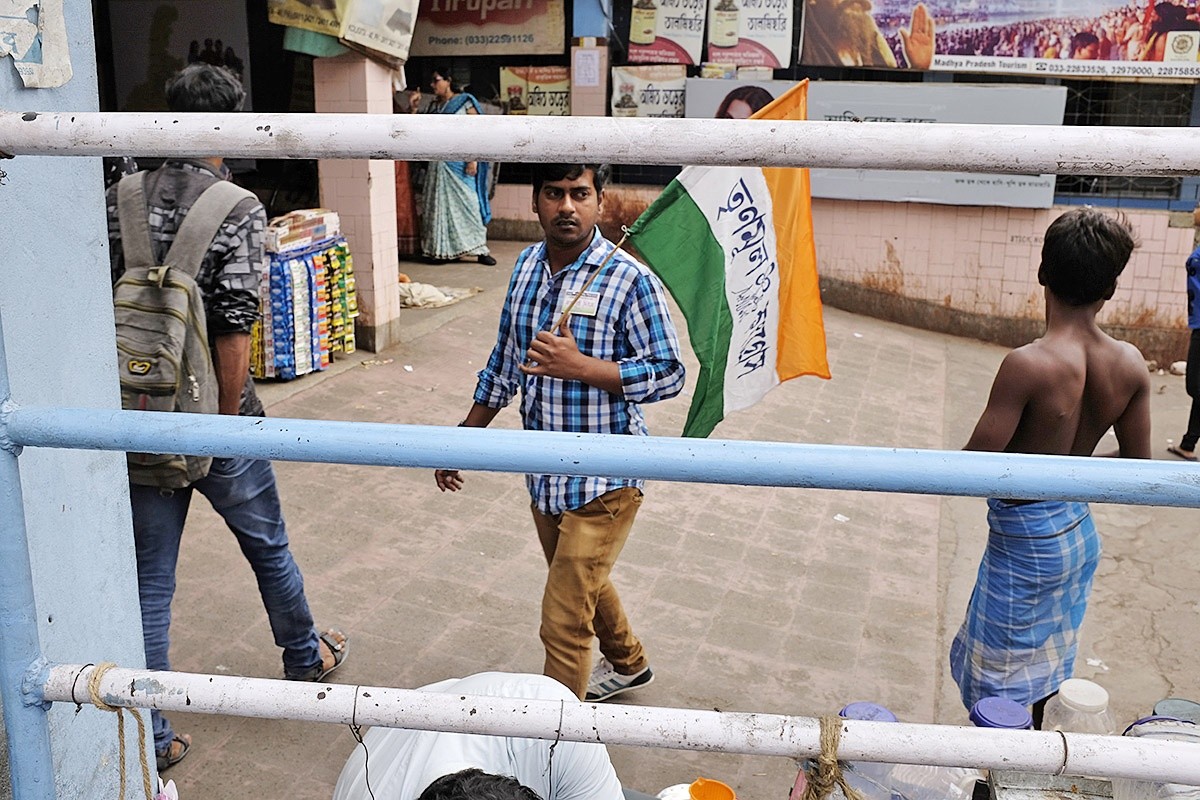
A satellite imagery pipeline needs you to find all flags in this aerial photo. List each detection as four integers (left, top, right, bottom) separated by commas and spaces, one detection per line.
624, 79, 832, 438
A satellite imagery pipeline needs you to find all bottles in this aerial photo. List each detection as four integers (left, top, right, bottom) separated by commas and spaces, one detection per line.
614, 94, 638, 117
837, 700, 899, 724
503, 96, 528, 116
710, 0, 739, 48
968, 696, 1033, 730
1041, 678, 1117, 736
629, 0, 658, 45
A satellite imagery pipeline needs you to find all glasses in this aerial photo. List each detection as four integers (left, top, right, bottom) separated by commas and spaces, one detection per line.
431, 76, 444, 83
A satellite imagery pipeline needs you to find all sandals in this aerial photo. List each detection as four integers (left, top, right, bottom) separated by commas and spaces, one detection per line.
282, 625, 349, 684
155, 732, 192, 772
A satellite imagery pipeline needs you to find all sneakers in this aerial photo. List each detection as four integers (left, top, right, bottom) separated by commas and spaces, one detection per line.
583, 656, 655, 704
477, 253, 497, 265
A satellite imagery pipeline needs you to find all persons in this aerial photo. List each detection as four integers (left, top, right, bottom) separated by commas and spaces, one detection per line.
802, 0, 936, 70
1165, 242, 1200, 461
870, 1, 1199, 62
435, 163, 686, 702
104, 62, 348, 772
392, 87, 421, 259
712, 87, 775, 121
331, 672, 631, 800
421, 68, 500, 266
951, 207, 1151, 737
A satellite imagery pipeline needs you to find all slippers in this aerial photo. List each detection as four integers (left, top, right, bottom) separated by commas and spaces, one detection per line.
1167, 445, 1199, 462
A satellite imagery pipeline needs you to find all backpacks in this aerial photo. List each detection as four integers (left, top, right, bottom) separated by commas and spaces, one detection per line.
112, 171, 259, 499
